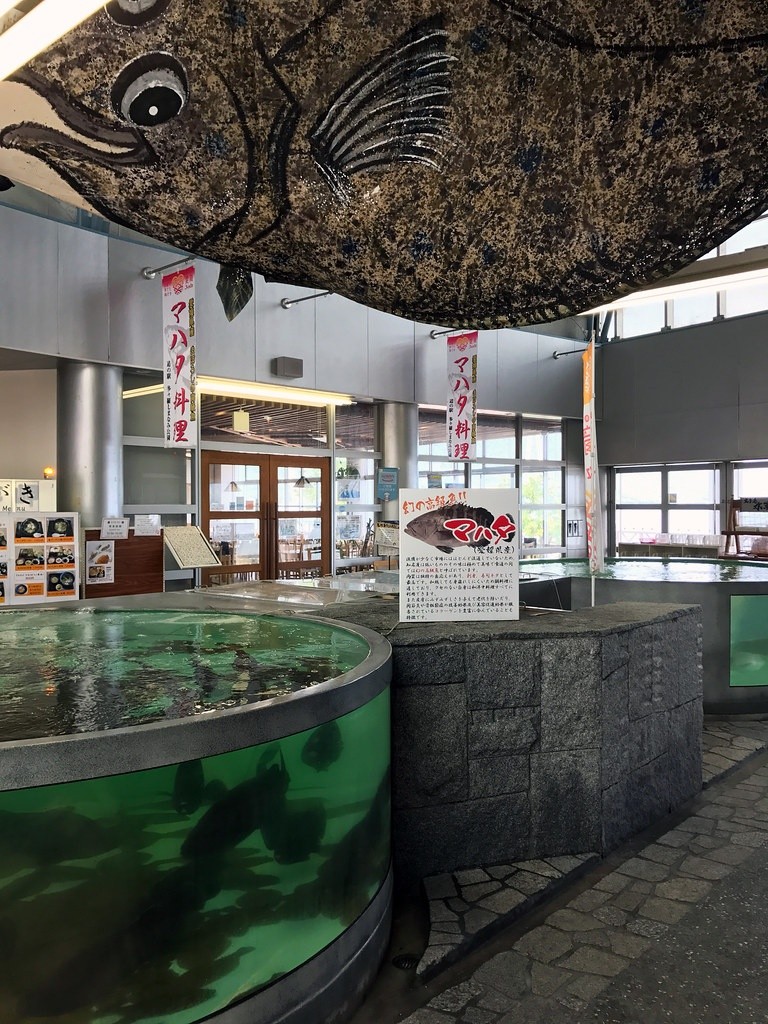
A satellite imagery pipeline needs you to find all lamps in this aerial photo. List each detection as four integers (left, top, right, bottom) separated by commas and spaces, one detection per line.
292, 468, 314, 488
224, 465, 241, 492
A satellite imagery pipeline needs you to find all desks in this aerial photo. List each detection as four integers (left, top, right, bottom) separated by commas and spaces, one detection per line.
286, 544, 321, 550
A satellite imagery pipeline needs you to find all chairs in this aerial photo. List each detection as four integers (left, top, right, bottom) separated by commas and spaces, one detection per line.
218, 534, 375, 585
523, 537, 537, 558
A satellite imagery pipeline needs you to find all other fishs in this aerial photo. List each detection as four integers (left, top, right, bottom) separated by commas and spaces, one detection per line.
0, 0, 768, 330
404, 504, 515, 554
0, 637, 392, 1024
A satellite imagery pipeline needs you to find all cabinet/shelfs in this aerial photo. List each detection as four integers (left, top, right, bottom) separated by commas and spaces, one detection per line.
618, 542, 723, 558
